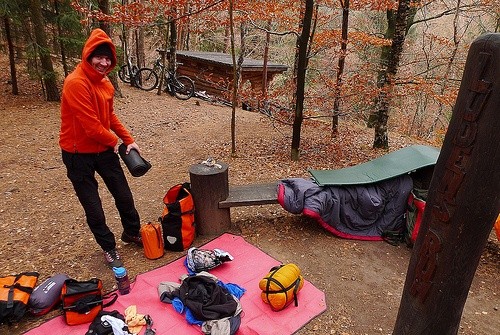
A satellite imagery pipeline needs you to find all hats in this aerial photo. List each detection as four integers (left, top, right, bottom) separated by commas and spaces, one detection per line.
88, 43, 113, 58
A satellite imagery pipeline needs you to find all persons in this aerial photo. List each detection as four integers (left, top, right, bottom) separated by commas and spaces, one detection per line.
59, 28, 153, 271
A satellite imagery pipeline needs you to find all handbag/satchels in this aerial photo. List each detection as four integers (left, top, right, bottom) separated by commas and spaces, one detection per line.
157, 183, 196, 252
0, 272, 40, 321
402, 188, 429, 247
59, 277, 104, 326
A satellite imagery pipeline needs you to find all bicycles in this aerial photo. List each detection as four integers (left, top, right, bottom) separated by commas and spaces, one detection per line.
118, 54, 142, 88
134, 54, 195, 100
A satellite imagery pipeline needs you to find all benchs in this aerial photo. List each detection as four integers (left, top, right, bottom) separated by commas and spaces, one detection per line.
220, 183, 278, 209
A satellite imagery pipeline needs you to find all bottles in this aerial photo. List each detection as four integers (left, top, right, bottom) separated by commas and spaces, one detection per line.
112, 267, 130, 296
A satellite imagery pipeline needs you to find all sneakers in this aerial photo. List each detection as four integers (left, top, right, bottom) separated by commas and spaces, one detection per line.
121, 232, 143, 245
104, 249, 122, 269
